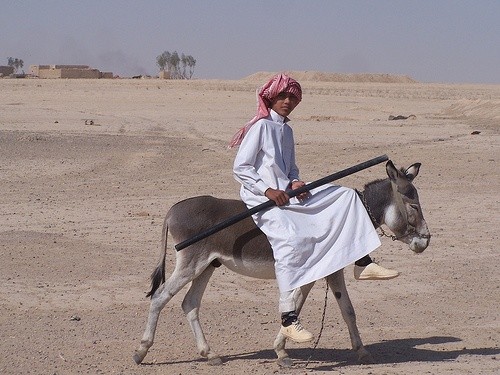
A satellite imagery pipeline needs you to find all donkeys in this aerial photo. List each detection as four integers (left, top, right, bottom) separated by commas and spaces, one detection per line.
132, 158, 431, 364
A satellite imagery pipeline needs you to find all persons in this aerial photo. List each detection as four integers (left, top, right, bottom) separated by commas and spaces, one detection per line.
227, 71, 401, 343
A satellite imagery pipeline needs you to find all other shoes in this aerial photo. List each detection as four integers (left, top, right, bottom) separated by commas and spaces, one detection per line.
353, 258, 399, 279
280, 321, 315, 342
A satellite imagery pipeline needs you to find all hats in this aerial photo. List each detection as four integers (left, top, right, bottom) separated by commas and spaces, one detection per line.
226, 73, 303, 149
